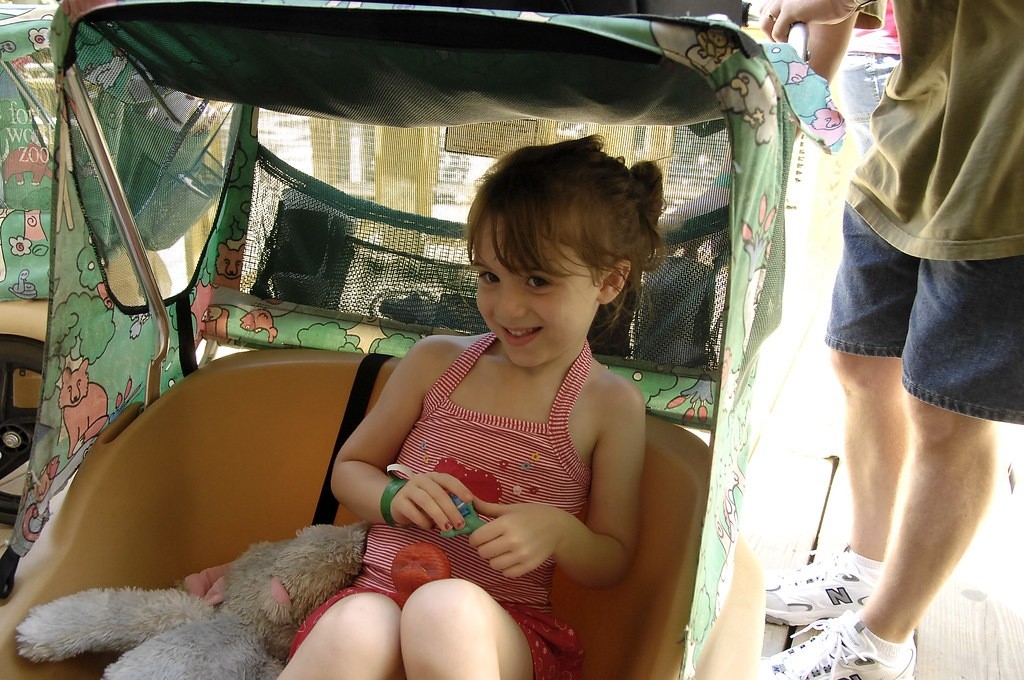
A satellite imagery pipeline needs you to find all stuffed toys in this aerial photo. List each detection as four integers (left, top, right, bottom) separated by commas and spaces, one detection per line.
16, 520, 370, 680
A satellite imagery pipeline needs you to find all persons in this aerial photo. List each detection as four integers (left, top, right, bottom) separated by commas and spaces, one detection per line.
757, 0, 1024, 680
279, 132, 663, 680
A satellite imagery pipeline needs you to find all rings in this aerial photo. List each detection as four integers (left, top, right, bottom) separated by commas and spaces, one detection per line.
765, 10, 776, 21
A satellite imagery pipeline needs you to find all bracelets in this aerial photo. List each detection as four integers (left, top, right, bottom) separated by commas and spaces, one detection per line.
380, 464, 413, 527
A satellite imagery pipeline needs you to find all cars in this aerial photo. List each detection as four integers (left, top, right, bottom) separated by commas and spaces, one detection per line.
433, 181, 464, 207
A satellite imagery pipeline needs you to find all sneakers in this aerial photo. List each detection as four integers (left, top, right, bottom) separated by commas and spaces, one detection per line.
764, 548, 877, 626
761, 610, 917, 680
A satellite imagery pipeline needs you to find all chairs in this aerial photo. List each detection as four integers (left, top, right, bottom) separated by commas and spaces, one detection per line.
0, 351, 709, 680
